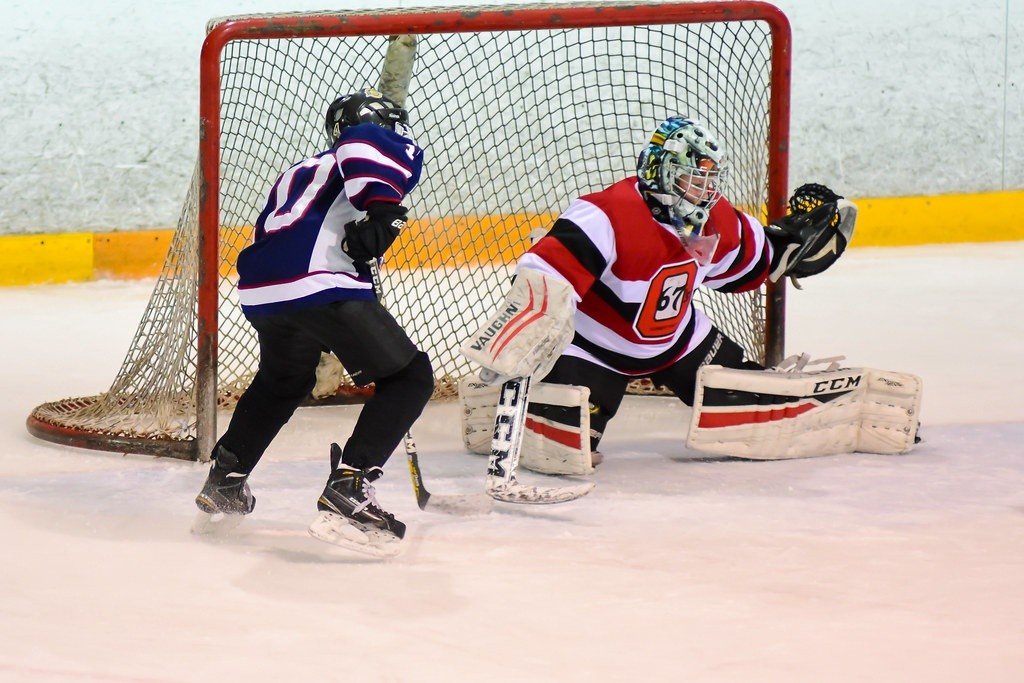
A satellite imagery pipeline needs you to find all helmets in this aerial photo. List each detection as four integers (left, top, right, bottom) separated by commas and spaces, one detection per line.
635, 116, 727, 239
325, 92, 414, 150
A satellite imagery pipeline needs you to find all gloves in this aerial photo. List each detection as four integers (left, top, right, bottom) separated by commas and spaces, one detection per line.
346, 203, 408, 261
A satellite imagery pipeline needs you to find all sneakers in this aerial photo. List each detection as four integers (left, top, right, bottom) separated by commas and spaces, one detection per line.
308, 443, 405, 556
191, 446, 256, 534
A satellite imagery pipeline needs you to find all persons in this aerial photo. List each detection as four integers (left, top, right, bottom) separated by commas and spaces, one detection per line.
195, 89, 437, 559
459, 116, 923, 475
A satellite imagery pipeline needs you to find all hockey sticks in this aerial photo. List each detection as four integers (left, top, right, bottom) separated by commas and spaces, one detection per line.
340, 219, 498, 518
483, 374, 597, 506
